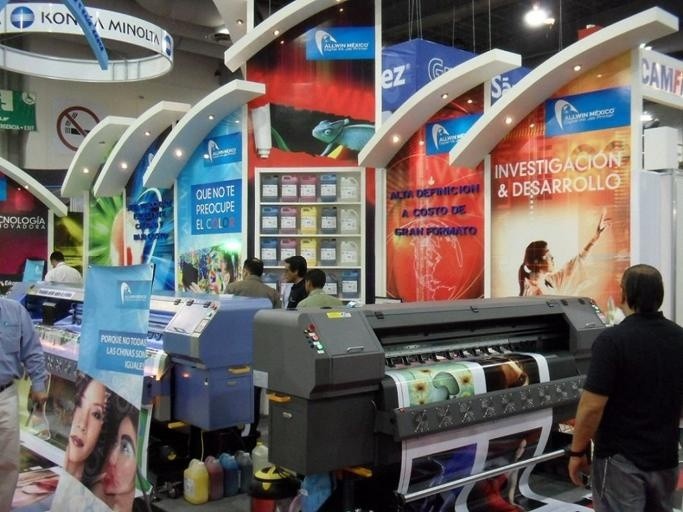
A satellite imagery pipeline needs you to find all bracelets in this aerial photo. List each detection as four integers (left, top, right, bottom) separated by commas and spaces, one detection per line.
565, 447, 587, 458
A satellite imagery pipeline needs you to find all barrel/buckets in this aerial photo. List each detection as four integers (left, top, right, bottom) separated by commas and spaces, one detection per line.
300, 206, 317, 233
300, 239, 317, 267
280, 206, 298, 233
320, 173, 337, 202
260, 239, 278, 266
340, 209, 359, 233
320, 239, 337, 265
261, 206, 279, 234
281, 175, 298, 201
322, 271, 339, 297
340, 241, 358, 266
261, 175, 278, 202
204, 455, 224, 502
320, 206, 338, 234
261, 272, 279, 292
252, 441, 268, 475
183, 459, 210, 504
235, 450, 253, 492
340, 177, 360, 201
341, 270, 360, 297
219, 453, 239, 497
280, 239, 297, 264
299, 175, 316, 201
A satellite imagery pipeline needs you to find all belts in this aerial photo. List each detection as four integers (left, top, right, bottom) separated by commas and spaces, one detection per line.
0, 380, 14, 395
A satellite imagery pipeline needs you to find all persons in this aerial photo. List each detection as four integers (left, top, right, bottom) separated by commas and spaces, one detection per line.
61, 373, 114, 490
0, 298, 49, 511
224, 257, 281, 309
296, 268, 343, 308
567, 263, 682, 512
519, 208, 612, 298
90, 413, 137, 512
483, 438, 529, 511
220, 253, 235, 287
44, 251, 81, 282
285, 256, 308, 308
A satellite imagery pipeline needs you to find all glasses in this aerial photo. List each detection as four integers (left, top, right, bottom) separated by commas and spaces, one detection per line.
282, 266, 293, 274
